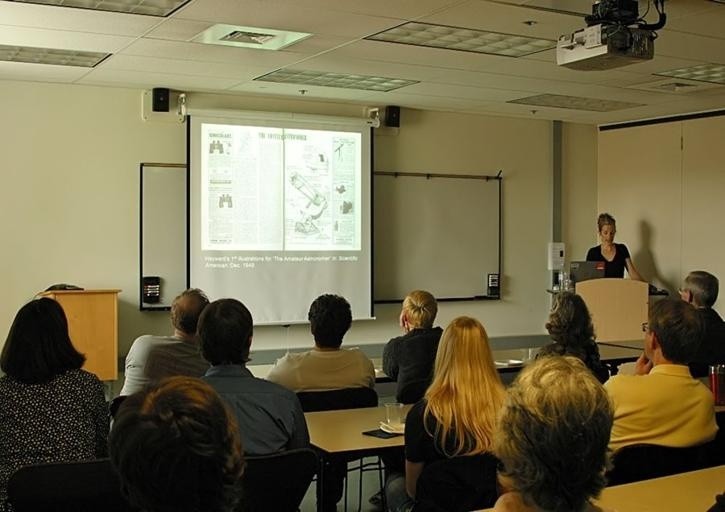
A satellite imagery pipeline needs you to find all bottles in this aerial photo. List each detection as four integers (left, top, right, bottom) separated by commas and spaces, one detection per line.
708, 363, 725, 406
558, 262, 569, 291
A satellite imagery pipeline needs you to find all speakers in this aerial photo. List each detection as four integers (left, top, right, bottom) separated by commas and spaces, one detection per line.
152, 88, 169, 113
384, 105, 400, 128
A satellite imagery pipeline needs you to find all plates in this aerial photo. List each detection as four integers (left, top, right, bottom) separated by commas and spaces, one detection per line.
379, 423, 406, 435
493, 359, 525, 366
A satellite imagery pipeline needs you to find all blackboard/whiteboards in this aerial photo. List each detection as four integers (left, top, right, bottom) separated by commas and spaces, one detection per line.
139, 163, 503, 312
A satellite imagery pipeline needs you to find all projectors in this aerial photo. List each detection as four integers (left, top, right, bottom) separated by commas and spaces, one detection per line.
556, 0, 666, 71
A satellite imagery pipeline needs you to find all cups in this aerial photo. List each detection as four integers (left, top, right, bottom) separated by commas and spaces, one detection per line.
563, 279, 575, 294
383, 402, 404, 427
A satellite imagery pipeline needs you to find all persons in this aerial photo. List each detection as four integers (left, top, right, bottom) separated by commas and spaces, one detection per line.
600, 296, 721, 459
533, 291, 609, 384
264, 294, 376, 511
586, 213, 643, 280
382, 290, 443, 400
195, 299, 310, 456
120, 289, 209, 396
109, 376, 248, 510
1, 298, 109, 511
475, 355, 615, 512
680, 271, 725, 378
385, 317, 508, 512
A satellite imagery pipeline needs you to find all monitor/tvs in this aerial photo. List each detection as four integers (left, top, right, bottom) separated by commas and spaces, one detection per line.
570, 261, 605, 287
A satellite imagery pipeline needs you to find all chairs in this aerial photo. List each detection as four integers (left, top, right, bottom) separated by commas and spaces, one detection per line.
8, 461, 117, 511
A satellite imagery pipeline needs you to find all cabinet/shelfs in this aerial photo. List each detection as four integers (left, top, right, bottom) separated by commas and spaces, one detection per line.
39, 289, 123, 382
